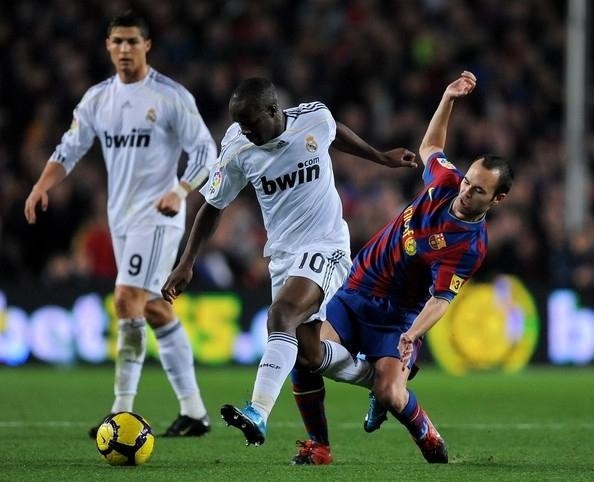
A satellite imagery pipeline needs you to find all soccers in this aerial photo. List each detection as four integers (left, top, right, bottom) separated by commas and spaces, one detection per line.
96, 412, 154, 467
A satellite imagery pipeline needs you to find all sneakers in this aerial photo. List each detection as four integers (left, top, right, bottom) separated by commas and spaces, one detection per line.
364, 393, 388, 433
87, 415, 113, 439
220, 401, 268, 447
161, 413, 212, 438
291, 439, 333, 465
406, 409, 448, 465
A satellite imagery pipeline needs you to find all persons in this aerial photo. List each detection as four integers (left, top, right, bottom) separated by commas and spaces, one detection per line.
288, 70, 514, 466
0, 1, 592, 305
24, 11, 211, 436
160, 77, 417, 444
